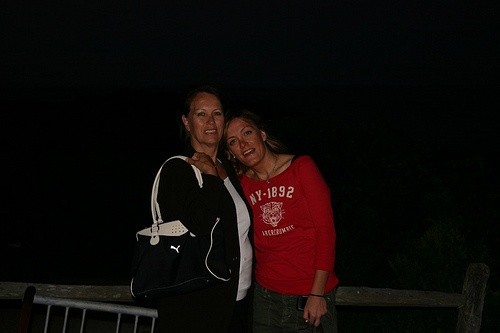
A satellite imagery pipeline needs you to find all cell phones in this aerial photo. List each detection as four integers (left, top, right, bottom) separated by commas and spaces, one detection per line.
297, 296, 309, 311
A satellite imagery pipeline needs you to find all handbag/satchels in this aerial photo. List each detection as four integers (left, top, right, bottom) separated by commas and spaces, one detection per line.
130, 156, 231, 302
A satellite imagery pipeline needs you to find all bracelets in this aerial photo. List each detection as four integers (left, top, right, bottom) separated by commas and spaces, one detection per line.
308, 292, 326, 298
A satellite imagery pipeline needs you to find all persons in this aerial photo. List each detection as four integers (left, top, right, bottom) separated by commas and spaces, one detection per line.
155, 85, 256, 333
220, 108, 339, 333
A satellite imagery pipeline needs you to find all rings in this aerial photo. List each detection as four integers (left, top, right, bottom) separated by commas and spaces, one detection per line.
315, 320, 321, 324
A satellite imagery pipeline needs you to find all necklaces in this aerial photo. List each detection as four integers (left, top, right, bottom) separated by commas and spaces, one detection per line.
254, 153, 278, 184
